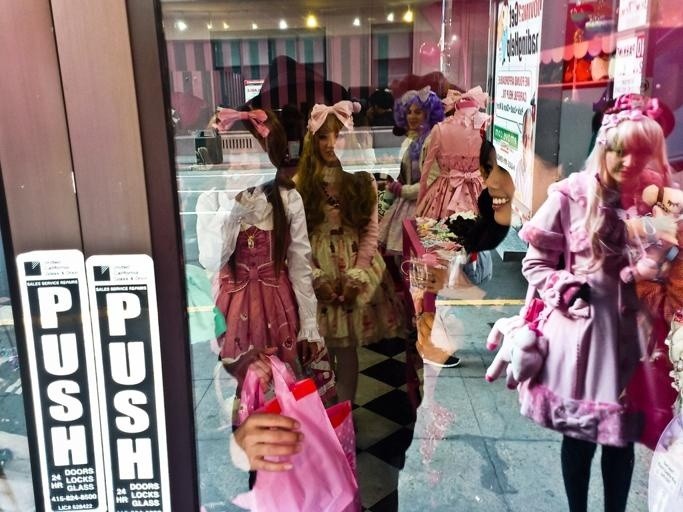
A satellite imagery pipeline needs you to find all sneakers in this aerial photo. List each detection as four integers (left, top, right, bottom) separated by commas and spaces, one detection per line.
422, 343, 461, 369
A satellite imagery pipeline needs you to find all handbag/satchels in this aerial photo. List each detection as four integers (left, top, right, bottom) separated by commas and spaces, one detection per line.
224, 381, 364, 512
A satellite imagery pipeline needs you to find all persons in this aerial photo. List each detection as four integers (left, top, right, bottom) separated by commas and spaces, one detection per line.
183, 54, 683, 512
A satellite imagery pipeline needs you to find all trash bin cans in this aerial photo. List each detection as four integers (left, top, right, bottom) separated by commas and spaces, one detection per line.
195, 127, 223, 165
